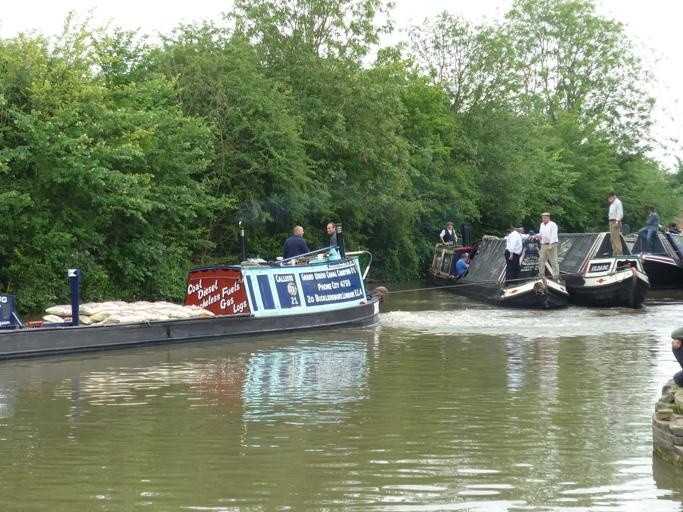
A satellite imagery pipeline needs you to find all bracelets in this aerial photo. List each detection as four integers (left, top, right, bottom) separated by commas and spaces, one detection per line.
615, 221, 619, 224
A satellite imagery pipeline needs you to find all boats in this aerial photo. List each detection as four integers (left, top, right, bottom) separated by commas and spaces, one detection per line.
426, 224, 683, 309
0, 222, 381, 360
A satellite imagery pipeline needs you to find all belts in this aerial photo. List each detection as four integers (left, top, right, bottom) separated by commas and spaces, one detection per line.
545, 242, 558, 245
609, 219, 621, 221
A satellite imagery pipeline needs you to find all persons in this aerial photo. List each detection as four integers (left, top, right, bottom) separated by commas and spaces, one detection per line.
528, 212, 559, 280
322, 221, 345, 260
503, 223, 530, 287
439, 221, 458, 245
606, 190, 624, 255
636, 204, 660, 253
455, 252, 472, 273
280, 225, 311, 268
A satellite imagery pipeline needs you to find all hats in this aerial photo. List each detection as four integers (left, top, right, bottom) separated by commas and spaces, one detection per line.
541, 212, 550, 216
